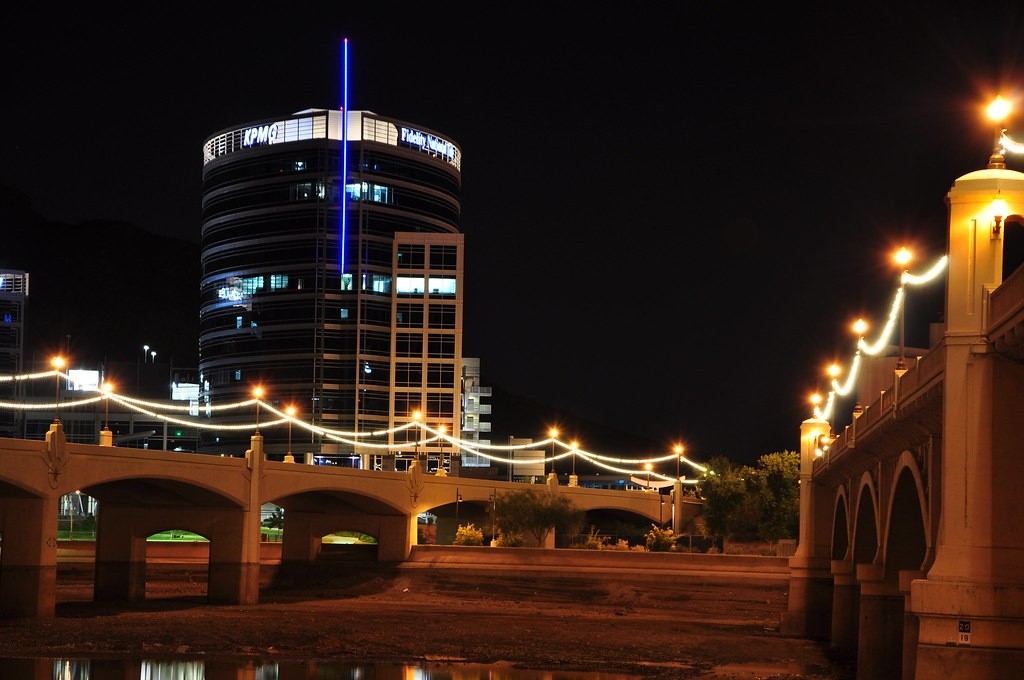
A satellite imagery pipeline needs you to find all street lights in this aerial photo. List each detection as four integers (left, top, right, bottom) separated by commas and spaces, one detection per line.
50, 357, 64, 424
252, 387, 262, 436
549, 429, 557, 473
645, 463, 653, 490
438, 426, 446, 469
571, 442, 578, 475
675, 445, 683, 480
455, 487, 464, 541
852, 317, 867, 409
982, 93, 1015, 169
659, 490, 666, 527
892, 245, 913, 370
413, 412, 422, 460
488, 489, 497, 541
285, 407, 295, 456
102, 383, 113, 431
